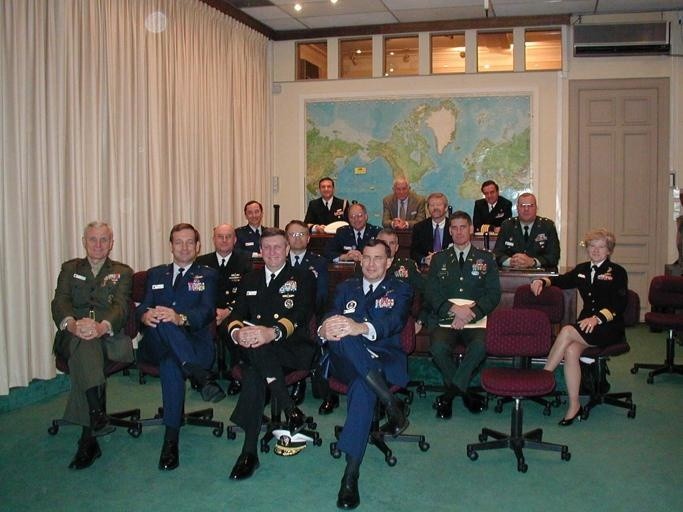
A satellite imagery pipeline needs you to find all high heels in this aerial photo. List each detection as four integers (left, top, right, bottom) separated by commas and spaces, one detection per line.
558, 404, 583, 427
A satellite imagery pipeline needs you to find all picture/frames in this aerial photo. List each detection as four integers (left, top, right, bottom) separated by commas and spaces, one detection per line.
296, 84, 540, 229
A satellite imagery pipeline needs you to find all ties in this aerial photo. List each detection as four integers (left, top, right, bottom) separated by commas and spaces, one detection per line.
523, 225, 530, 251
173, 268, 184, 287
590, 265, 597, 283
220, 258, 224, 277
356, 232, 361, 246
433, 224, 441, 253
318, 395, 339, 415
255, 229, 260, 242
399, 201, 405, 221
324, 202, 328, 223
267, 274, 276, 287
458, 252, 464, 272
365, 284, 373, 299
294, 256, 299, 266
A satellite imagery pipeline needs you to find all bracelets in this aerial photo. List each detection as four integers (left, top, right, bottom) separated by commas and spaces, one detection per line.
178, 312, 188, 327
530, 258, 537, 269
272, 325, 280, 340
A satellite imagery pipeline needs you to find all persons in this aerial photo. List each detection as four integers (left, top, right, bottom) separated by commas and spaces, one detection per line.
491, 192, 562, 271
313, 237, 416, 509
471, 178, 512, 233
223, 227, 321, 481
422, 210, 503, 420
193, 174, 453, 414
530, 229, 628, 426
130, 221, 226, 472
49, 221, 136, 471
190, 224, 255, 396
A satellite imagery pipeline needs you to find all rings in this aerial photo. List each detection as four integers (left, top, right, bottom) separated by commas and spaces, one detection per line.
588, 323, 592, 327
340, 327, 344, 331
90, 329, 94, 336
255, 338, 258, 344
242, 339, 249, 345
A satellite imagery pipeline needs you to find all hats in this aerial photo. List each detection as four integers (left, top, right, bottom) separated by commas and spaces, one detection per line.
271, 429, 313, 455
324, 220, 348, 235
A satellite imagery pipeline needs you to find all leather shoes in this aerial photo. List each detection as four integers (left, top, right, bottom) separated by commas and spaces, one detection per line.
285, 406, 306, 436
291, 379, 306, 405
384, 397, 410, 437
462, 395, 485, 413
336, 475, 359, 508
200, 378, 225, 403
228, 452, 259, 480
228, 378, 241, 396
158, 440, 179, 469
88, 411, 115, 436
433, 395, 452, 419
68, 438, 101, 469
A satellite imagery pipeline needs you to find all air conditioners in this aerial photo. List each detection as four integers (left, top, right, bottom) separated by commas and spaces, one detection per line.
572, 20, 671, 59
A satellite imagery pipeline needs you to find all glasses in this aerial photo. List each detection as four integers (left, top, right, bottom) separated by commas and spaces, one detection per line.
518, 204, 535, 208
286, 232, 307, 238
